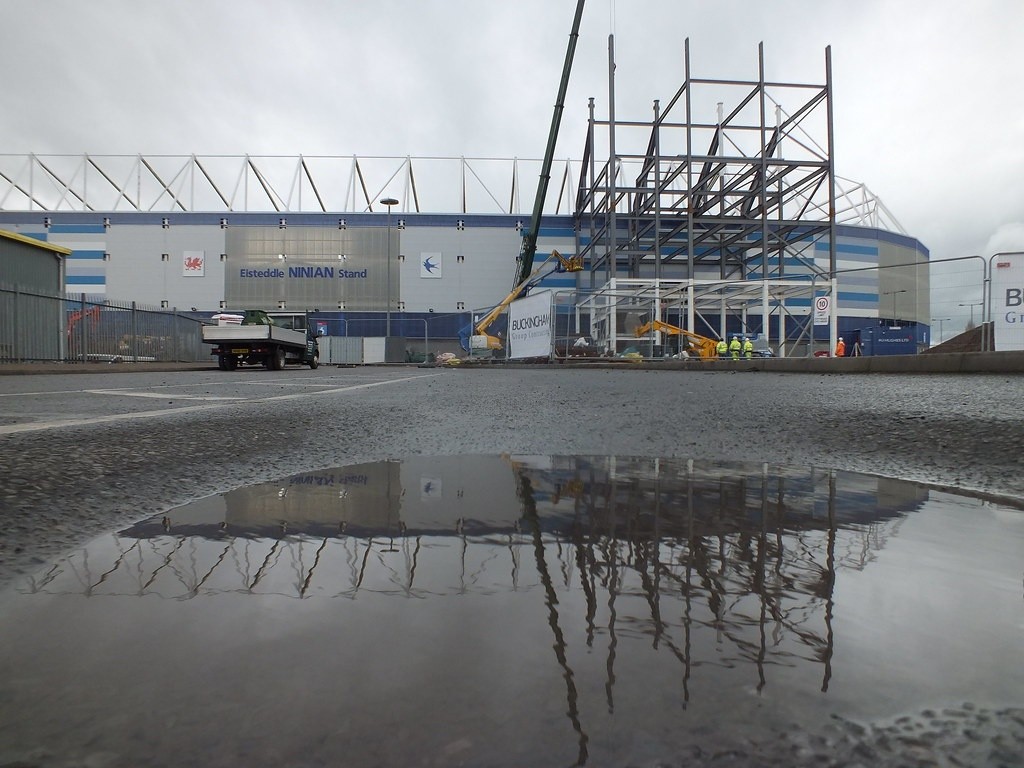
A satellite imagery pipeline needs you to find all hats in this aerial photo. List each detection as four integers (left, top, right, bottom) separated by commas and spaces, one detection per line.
839, 338, 843, 340
720, 338, 724, 341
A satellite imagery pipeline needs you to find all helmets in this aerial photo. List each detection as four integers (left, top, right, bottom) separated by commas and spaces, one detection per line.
745, 338, 749, 341
733, 337, 737, 339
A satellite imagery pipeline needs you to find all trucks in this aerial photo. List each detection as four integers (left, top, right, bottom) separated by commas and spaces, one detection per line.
201, 308, 319, 371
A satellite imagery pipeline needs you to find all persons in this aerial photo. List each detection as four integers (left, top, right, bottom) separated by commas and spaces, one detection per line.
730, 336, 741, 360
835, 337, 845, 357
716, 338, 727, 360
744, 338, 753, 360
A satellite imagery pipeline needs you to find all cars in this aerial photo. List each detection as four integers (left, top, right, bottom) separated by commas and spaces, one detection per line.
437, 353, 456, 361
805, 350, 839, 358
742, 350, 775, 358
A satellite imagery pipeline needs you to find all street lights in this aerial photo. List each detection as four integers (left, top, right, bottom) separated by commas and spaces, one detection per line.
379, 198, 399, 337
932, 319, 951, 344
882, 290, 906, 327
959, 302, 983, 330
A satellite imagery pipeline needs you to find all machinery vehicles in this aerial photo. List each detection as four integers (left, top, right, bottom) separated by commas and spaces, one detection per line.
457, 249, 585, 359
634, 320, 720, 361
67, 300, 110, 344
